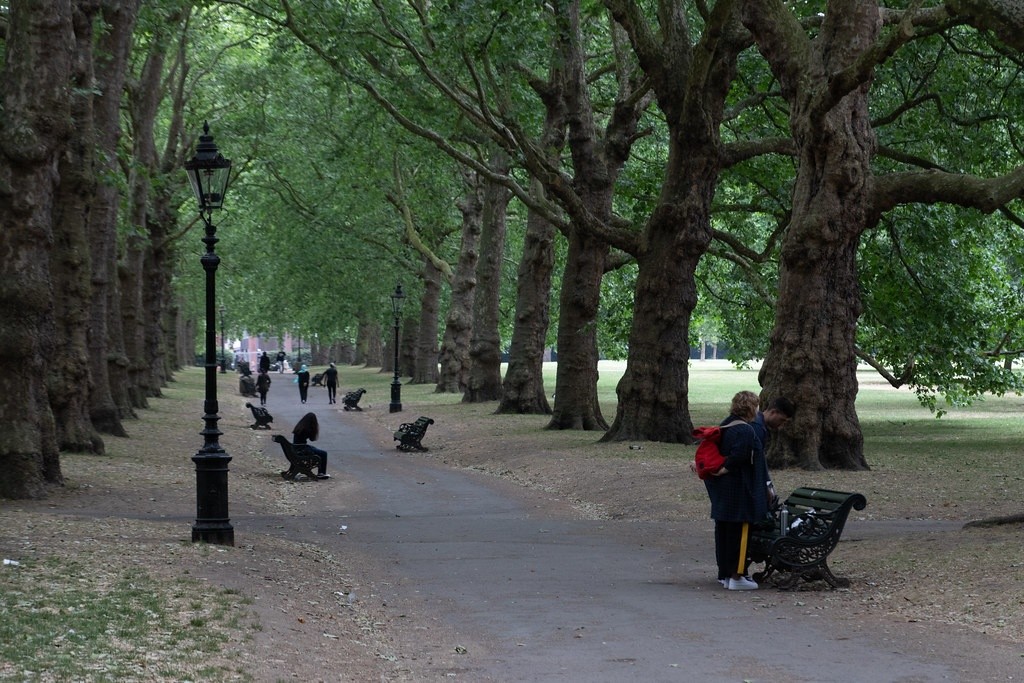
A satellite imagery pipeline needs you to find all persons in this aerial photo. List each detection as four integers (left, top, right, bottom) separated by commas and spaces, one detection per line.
323, 362, 339, 403
703, 391, 775, 591
298, 364, 309, 403
277, 347, 286, 374
256, 368, 271, 406
750, 396, 793, 450
260, 352, 269, 372
293, 412, 330, 478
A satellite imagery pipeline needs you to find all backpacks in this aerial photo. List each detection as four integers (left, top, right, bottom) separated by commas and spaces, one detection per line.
691, 420, 755, 480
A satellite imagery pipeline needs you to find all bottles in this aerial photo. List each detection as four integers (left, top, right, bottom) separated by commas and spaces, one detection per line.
780, 505, 788, 536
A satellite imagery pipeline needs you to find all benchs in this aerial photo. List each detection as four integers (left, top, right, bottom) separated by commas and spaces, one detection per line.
311, 374, 325, 386
246, 403, 273, 429
343, 388, 366, 411
392, 417, 434, 449
272, 434, 322, 480
240, 361, 257, 396
747, 487, 866, 590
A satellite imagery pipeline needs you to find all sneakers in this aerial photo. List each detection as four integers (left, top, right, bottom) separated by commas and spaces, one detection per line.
724, 577, 730, 589
728, 576, 759, 590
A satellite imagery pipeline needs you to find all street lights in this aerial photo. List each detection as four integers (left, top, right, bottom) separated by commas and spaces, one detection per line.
389, 282, 402, 414
181, 118, 234, 546
218, 305, 226, 373
